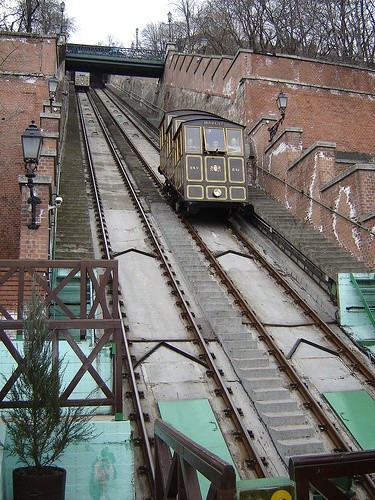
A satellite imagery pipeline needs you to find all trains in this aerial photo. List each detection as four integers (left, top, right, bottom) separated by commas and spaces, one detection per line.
158, 108, 249, 217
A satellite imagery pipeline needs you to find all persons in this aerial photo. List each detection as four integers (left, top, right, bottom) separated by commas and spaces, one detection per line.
84, 444, 118, 500
228, 136, 241, 153
186, 137, 199, 150
207, 139, 223, 150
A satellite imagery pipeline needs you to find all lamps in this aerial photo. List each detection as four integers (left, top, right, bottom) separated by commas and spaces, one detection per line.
46, 73, 59, 112
20, 120, 44, 229
268, 91, 288, 142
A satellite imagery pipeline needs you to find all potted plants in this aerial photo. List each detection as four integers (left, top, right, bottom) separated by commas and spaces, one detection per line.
0, 272, 111, 500
